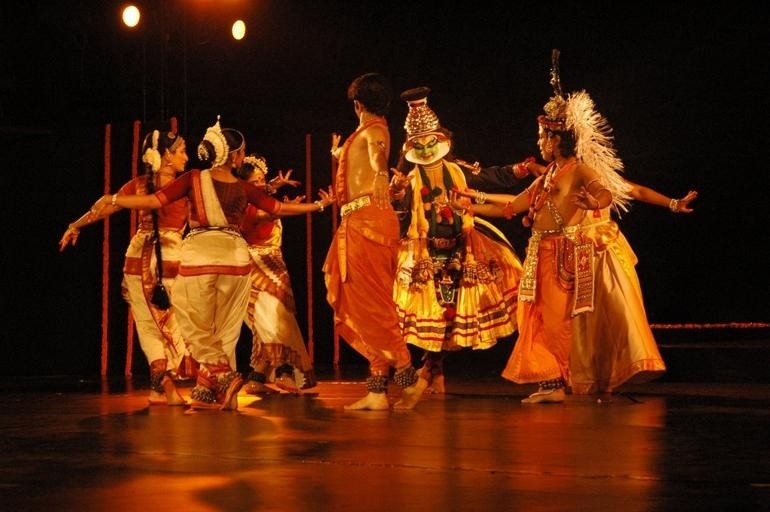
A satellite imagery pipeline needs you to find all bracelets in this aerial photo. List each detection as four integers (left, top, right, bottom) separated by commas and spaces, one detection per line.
264, 184, 277, 195
112, 194, 118, 207
669, 198, 679, 211
595, 199, 600, 212
476, 190, 487, 205
315, 201, 324, 213
389, 184, 406, 201
67, 224, 80, 235
456, 201, 471, 217
374, 169, 390, 177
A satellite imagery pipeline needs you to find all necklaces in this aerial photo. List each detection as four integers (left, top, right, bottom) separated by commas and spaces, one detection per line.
358, 118, 377, 125
158, 171, 176, 179
551, 157, 575, 183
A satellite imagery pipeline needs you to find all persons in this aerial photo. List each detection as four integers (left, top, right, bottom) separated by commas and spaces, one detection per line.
452, 158, 699, 404
236, 153, 316, 397
85, 128, 337, 408
58, 129, 190, 406
323, 74, 429, 410
448, 97, 666, 404
389, 87, 546, 394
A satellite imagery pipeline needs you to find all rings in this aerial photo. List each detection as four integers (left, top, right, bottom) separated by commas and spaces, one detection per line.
91, 209, 96, 213
